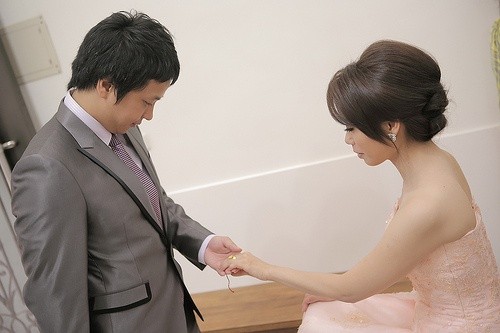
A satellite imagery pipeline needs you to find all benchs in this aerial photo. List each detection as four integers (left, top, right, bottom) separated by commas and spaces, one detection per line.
189, 270, 414, 333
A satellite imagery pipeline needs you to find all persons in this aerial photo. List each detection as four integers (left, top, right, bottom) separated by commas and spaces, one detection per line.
10, 10, 242, 333
220, 40, 500, 333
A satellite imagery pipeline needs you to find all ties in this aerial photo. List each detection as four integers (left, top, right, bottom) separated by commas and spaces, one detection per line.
108, 134, 163, 230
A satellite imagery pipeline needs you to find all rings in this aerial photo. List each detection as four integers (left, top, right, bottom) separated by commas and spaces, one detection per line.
228, 255, 236, 259
